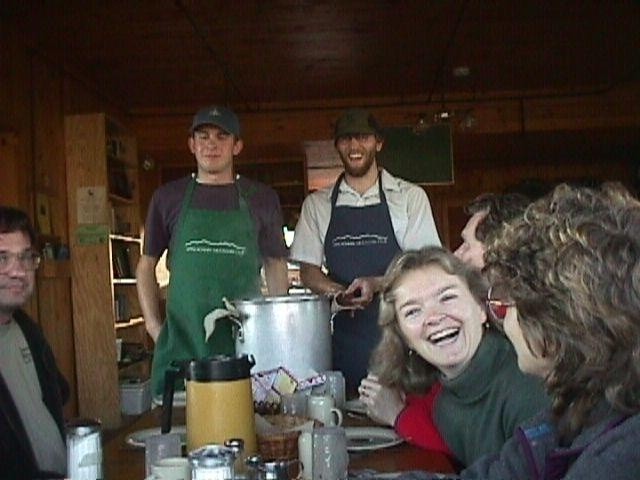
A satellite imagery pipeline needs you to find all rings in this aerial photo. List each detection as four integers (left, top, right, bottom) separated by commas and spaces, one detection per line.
361, 385, 366, 394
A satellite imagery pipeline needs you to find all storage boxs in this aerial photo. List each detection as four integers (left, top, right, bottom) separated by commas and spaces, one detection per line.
117, 378, 151, 416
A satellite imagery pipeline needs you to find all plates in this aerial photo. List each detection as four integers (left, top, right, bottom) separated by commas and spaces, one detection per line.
125, 424, 188, 447
343, 426, 404, 451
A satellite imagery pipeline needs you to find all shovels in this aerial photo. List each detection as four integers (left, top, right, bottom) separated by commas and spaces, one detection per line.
334, 291, 354, 310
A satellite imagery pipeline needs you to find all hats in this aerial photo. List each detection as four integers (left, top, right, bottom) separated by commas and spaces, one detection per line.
192, 106, 240, 138
333, 110, 378, 136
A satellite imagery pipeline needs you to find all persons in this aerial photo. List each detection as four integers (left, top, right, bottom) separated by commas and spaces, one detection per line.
346, 178, 640, 479
371, 241, 552, 468
0, 206, 72, 480
356, 188, 531, 462
134, 103, 291, 409
291, 107, 447, 400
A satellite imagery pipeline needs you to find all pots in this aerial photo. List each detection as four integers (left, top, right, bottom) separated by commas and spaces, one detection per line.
221, 290, 337, 380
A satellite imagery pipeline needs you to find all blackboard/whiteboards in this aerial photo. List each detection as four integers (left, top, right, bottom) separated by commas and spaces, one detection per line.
378, 122, 455, 186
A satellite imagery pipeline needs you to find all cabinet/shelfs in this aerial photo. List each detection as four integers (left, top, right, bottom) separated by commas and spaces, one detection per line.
157, 157, 307, 231
63, 110, 161, 431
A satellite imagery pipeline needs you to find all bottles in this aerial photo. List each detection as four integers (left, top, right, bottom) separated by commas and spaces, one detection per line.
65, 417, 104, 480
188, 443, 236, 480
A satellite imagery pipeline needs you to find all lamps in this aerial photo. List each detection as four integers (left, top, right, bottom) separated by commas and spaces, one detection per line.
411, 96, 476, 137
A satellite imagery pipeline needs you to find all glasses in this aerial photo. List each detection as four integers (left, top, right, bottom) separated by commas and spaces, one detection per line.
486, 285, 517, 320
0, 249, 43, 274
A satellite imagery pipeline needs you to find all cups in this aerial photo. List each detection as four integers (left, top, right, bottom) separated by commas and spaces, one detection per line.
145, 433, 191, 480
297, 426, 349, 480
280, 393, 343, 426
